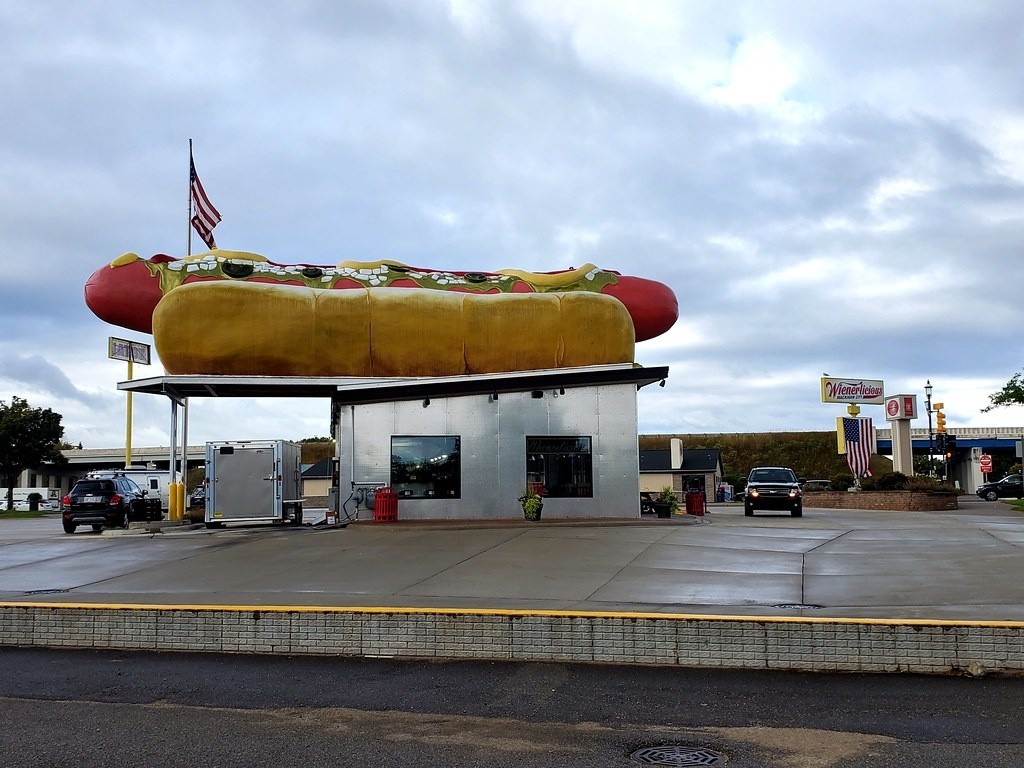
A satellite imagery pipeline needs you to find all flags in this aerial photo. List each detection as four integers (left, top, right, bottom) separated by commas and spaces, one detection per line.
842, 417, 873, 480
189, 154, 223, 251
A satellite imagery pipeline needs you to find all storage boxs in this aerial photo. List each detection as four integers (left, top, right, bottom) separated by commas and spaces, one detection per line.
326, 511, 336, 525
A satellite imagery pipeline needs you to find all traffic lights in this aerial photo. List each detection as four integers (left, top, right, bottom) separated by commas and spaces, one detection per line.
933, 403, 943, 410
945, 435, 956, 448
937, 413, 946, 433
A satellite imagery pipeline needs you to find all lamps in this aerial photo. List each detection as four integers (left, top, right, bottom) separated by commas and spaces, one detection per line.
532, 391, 543, 399
560, 384, 565, 395
553, 388, 558, 398
425, 395, 430, 406
659, 380, 665, 387
493, 389, 498, 400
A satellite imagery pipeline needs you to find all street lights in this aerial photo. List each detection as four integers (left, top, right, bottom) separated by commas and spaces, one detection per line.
924, 379, 934, 476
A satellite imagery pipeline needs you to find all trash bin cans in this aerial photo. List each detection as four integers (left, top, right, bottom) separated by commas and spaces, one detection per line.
374, 487, 398, 523
686, 494, 705, 516
717, 490, 724, 503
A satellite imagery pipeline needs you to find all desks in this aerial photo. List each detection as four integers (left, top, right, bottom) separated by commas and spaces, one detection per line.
640, 490, 683, 514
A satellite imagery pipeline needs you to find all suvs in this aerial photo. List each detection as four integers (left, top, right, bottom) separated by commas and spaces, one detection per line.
190, 485, 205, 506
62, 472, 150, 533
738, 467, 807, 516
38, 499, 52, 511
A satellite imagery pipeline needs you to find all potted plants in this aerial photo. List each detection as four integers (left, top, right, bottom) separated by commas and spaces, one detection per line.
517, 486, 544, 522
655, 485, 681, 518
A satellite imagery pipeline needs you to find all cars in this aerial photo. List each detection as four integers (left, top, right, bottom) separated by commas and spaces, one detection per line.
13, 502, 21, 510
0, 501, 8, 511
976, 474, 1024, 501
734, 492, 745, 503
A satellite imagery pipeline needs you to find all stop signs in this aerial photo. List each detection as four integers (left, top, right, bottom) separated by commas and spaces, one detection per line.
979, 455, 991, 466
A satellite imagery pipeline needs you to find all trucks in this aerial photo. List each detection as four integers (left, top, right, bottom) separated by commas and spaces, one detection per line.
205, 440, 307, 529
